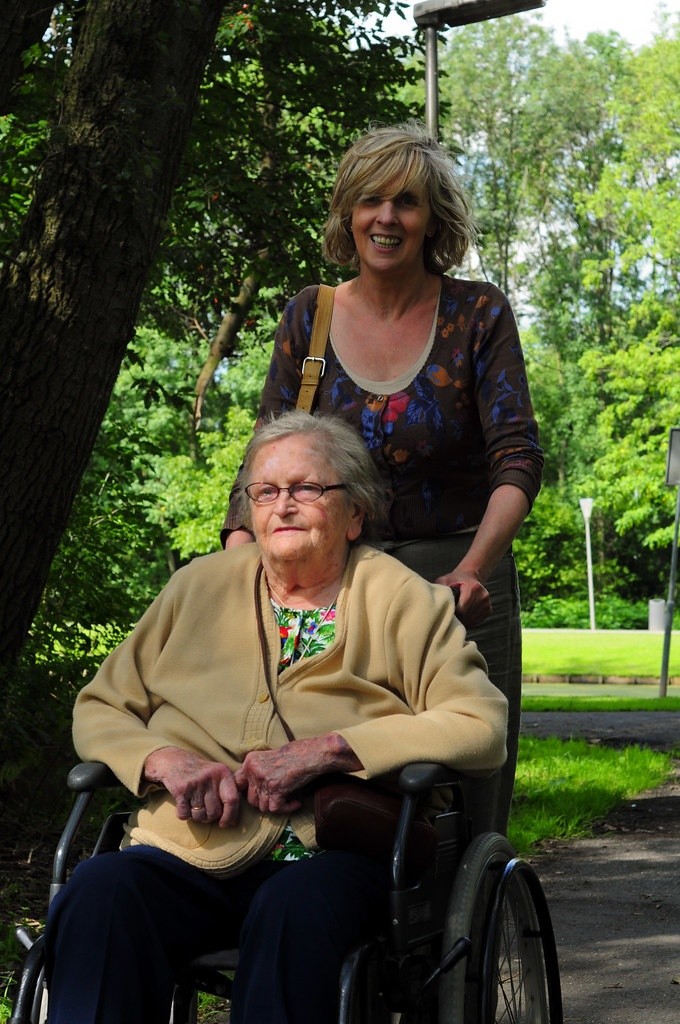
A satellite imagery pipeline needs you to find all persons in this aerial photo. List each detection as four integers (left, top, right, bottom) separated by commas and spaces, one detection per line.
220, 128, 545, 1024
44, 414, 508, 1024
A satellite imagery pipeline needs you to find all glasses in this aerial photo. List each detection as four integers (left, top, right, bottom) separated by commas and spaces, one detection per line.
245, 481, 354, 503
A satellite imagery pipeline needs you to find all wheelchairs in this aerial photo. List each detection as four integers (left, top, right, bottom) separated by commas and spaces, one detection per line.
9, 589, 572, 1024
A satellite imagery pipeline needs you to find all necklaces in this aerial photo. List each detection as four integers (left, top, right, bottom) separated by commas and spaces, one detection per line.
270, 586, 340, 661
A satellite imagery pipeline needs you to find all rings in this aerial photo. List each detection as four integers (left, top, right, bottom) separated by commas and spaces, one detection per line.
192, 806, 204, 810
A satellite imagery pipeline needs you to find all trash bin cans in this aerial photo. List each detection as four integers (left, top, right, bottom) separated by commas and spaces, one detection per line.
648, 598, 665, 632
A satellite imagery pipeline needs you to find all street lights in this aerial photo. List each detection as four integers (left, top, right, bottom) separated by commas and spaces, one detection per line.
580, 496, 598, 631
415, 0, 549, 144
